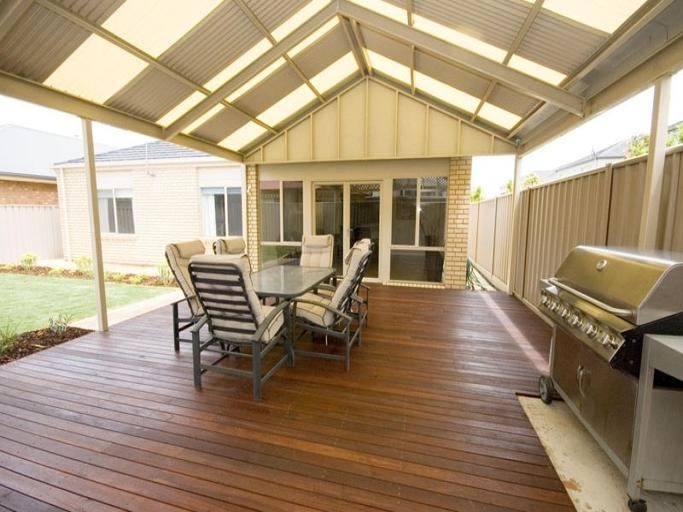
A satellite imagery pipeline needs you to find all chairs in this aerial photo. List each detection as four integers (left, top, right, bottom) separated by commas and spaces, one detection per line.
163, 232, 375, 401
